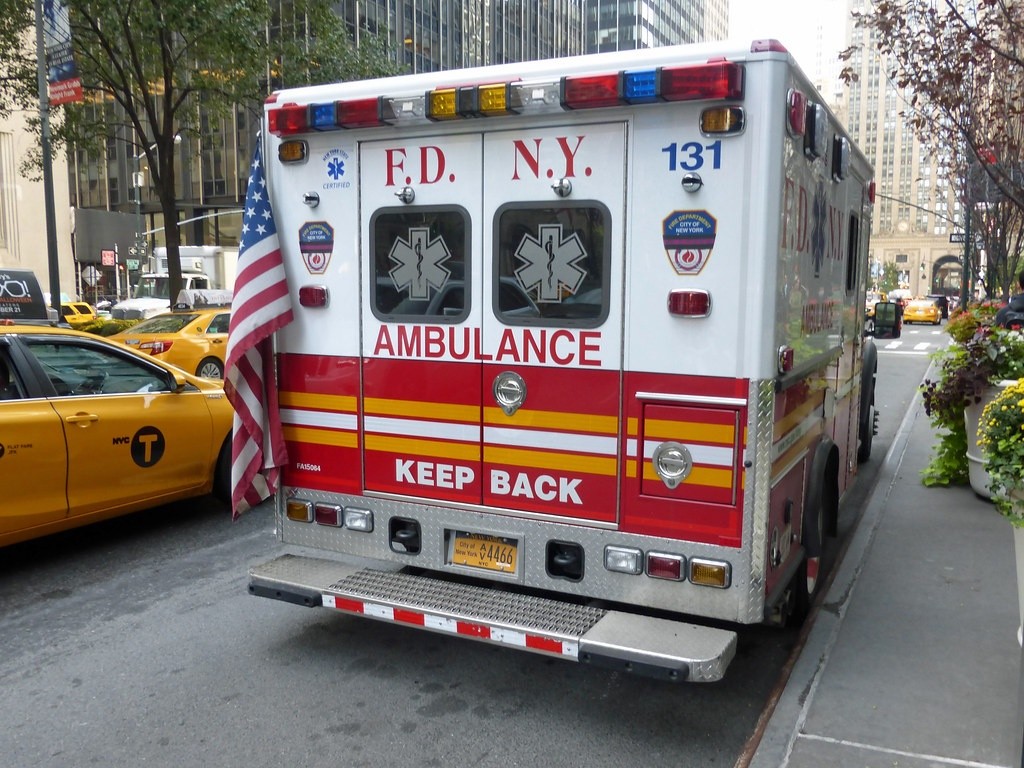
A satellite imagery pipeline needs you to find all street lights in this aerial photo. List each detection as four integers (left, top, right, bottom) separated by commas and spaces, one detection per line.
132, 133, 183, 296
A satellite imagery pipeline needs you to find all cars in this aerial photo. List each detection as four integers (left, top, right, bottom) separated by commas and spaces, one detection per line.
890, 288, 913, 305
172, 287, 234, 309
887, 294, 904, 315
47, 302, 98, 324
0, 319, 234, 551
902, 300, 943, 325
865, 289, 888, 318
105, 308, 232, 379
924, 294, 960, 318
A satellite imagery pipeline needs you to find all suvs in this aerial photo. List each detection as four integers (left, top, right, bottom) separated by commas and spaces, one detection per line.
111, 273, 213, 319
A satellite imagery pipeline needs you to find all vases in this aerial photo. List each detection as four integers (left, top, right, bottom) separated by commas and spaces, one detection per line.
964, 380, 1024, 502
1009, 489, 1024, 648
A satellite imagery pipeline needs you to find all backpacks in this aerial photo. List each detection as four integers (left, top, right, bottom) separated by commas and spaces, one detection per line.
1006, 303, 1024, 331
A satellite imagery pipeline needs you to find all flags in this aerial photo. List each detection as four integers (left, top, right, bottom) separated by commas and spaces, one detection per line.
223, 135, 294, 521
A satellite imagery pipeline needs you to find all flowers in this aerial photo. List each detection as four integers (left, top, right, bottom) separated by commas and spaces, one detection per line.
976, 378, 1024, 530
916, 323, 1024, 487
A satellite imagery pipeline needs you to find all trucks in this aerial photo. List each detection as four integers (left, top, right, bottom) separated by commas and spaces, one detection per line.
0, 269, 49, 319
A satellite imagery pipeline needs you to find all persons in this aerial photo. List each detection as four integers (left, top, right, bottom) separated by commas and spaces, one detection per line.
995, 269, 1024, 329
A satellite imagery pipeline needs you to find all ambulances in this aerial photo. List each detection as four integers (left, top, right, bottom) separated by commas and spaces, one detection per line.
242, 37, 903, 689
